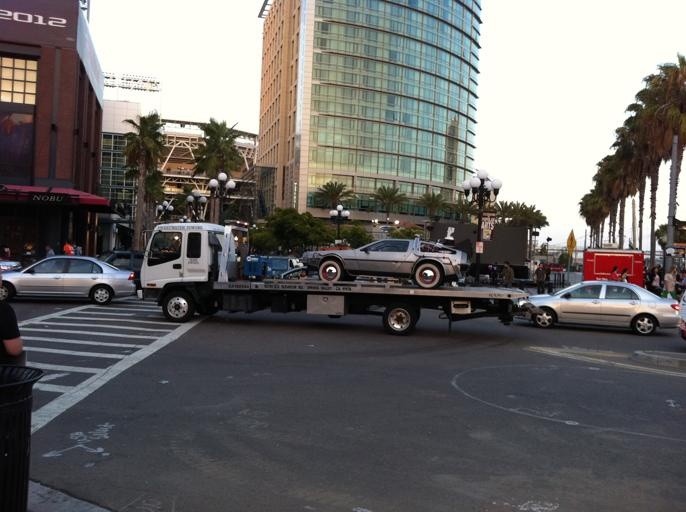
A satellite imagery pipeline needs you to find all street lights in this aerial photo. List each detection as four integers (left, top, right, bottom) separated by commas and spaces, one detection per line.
329, 205, 350, 240
461, 171, 502, 287
250, 224, 264, 248
157, 200, 174, 224
372, 217, 400, 239
209, 172, 236, 225
185, 190, 207, 222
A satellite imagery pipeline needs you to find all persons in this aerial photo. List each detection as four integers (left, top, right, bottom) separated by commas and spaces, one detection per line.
64, 240, 82, 256
0, 302, 25, 364
609, 264, 686, 302
502, 261, 514, 288
45, 245, 54, 257
535, 263, 546, 294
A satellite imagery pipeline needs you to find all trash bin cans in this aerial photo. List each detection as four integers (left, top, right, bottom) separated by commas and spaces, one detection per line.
0, 363, 46, 512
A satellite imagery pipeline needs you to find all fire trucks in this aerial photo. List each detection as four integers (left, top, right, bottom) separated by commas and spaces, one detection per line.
583, 244, 645, 294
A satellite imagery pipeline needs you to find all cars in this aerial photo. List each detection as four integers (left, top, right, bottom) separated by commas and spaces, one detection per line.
301, 237, 471, 289
512, 280, 681, 336
545, 263, 563, 272
0, 249, 144, 305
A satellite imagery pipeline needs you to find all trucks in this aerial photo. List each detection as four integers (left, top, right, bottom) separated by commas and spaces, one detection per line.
138, 223, 531, 335
